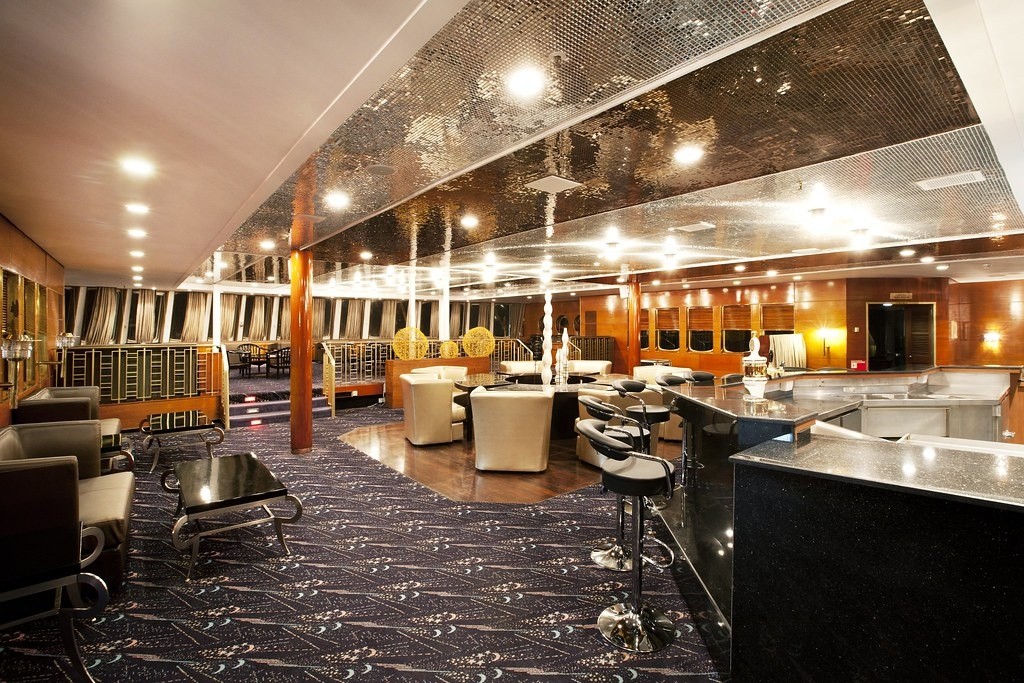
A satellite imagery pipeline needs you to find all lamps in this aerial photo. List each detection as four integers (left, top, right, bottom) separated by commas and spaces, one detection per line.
36, 332, 80, 378
0, 330, 37, 410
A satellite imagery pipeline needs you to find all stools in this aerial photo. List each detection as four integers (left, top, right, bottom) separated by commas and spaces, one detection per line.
576, 419, 677, 651
578, 394, 659, 573
614, 378, 669, 519
656, 370, 743, 386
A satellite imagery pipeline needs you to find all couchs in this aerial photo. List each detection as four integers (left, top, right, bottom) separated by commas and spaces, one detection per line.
20, 385, 132, 473
400, 373, 466, 445
410, 360, 612, 380
470, 386, 556, 473
632, 364, 693, 441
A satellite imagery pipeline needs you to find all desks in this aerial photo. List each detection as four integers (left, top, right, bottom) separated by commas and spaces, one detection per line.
139, 409, 224, 474
455, 369, 632, 441
243, 355, 276, 377
161, 452, 304, 583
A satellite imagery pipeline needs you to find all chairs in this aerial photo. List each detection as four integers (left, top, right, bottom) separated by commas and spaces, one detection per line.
238, 343, 270, 374
265, 347, 291, 378
217, 345, 251, 378
0, 420, 136, 683
